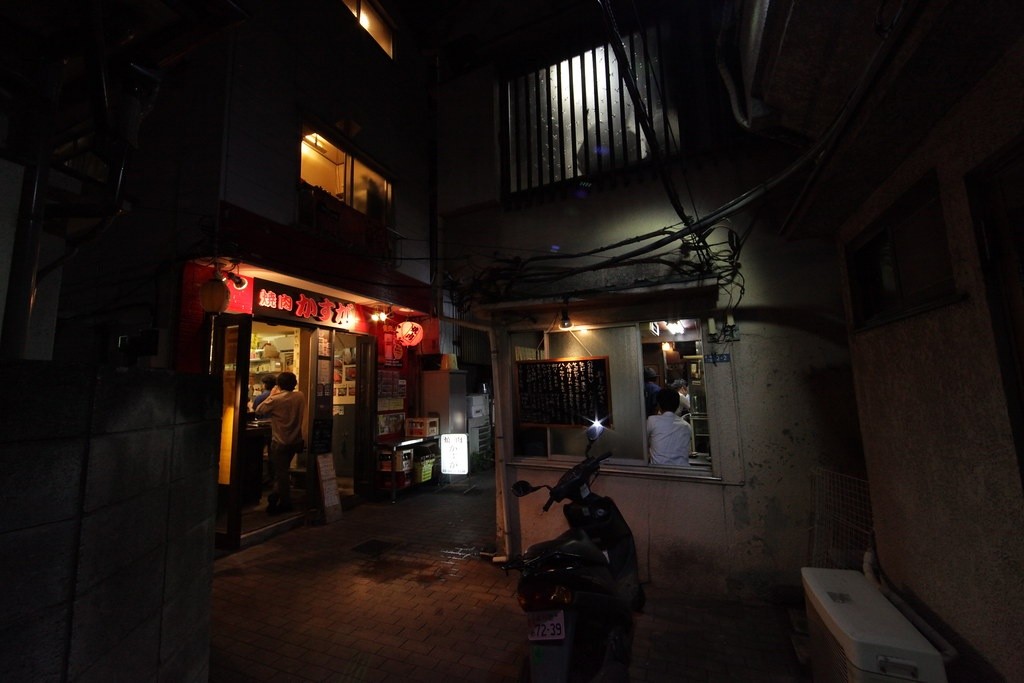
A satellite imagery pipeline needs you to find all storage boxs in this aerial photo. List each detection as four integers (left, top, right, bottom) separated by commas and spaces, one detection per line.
404, 417, 440, 438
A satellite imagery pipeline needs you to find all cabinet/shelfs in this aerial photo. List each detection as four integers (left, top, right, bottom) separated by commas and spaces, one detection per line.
248, 349, 293, 408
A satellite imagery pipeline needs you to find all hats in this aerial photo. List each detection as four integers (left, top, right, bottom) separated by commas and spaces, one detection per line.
644, 366, 660, 378
262, 374, 277, 385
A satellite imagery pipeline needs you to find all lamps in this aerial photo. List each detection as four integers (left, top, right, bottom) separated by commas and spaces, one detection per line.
227, 261, 248, 290
560, 309, 571, 327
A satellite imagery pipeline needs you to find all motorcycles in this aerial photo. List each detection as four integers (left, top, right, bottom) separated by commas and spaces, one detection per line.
500, 420, 648, 683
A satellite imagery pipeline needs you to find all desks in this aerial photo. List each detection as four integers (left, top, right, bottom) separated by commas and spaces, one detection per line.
376, 431, 441, 504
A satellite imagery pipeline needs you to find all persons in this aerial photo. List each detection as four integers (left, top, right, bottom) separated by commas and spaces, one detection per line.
644, 366, 690, 416
252, 372, 306, 514
647, 388, 693, 466
